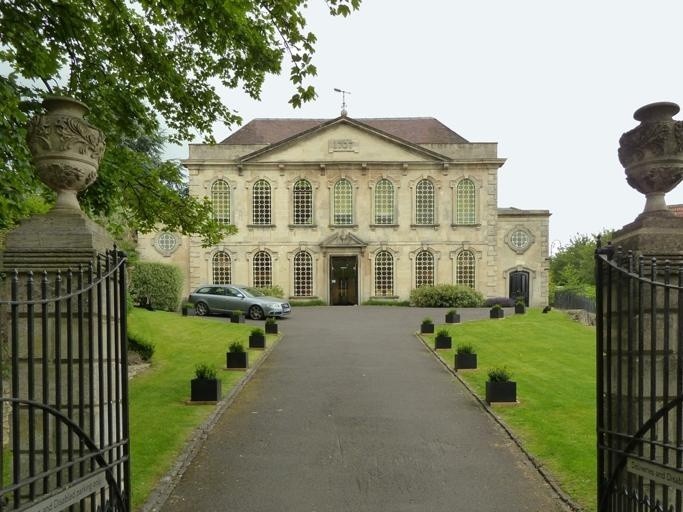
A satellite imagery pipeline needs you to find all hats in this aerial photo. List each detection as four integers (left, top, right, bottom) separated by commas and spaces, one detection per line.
188, 283, 292, 320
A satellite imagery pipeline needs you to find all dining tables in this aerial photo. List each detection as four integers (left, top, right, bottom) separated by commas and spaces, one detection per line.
490, 301, 524, 317
182, 303, 194, 315
249, 317, 277, 347
486, 364, 516, 403
192, 363, 221, 401
230, 309, 245, 324
422, 307, 459, 349
454, 342, 477, 369
227, 341, 248, 367
